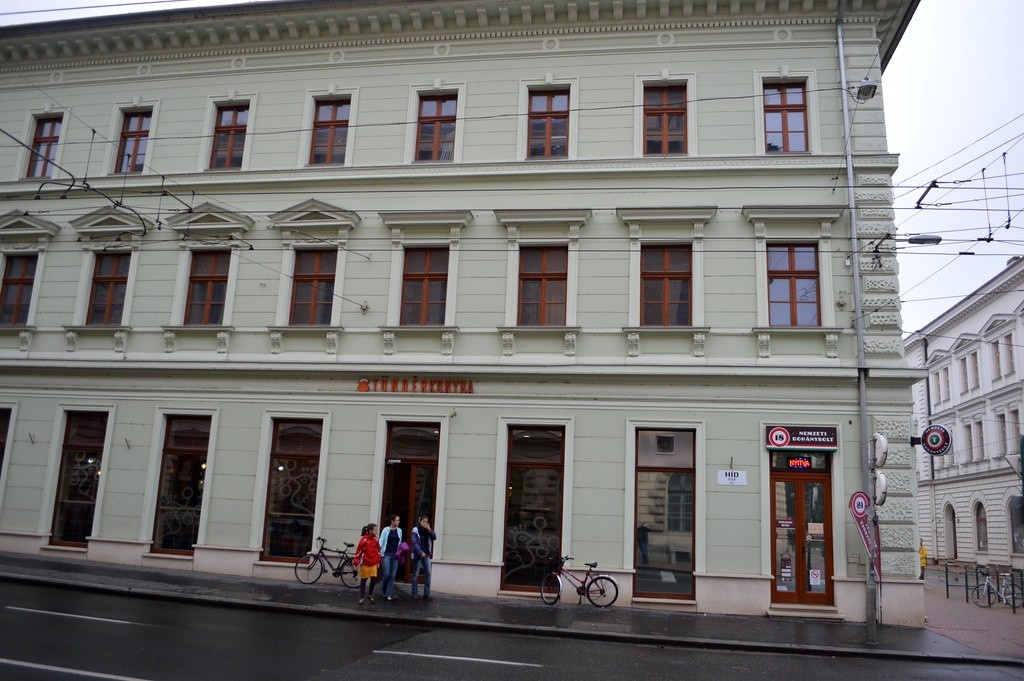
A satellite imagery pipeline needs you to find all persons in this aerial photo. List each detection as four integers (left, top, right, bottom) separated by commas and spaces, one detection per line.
353, 523, 382, 605
918, 538, 928, 580
379, 514, 402, 601
637, 521, 664, 565
785, 529, 795, 553
410, 514, 437, 601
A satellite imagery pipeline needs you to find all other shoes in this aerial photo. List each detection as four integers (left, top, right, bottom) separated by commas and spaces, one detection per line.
425, 595, 434, 599
413, 595, 421, 600
387, 596, 392, 600
368, 595, 375, 602
382, 593, 386, 598
358, 597, 365, 605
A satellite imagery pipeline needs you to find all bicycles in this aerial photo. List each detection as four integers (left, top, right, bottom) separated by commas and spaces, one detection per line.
294, 536, 369, 588
540, 555, 620, 607
971, 566, 1024, 607
